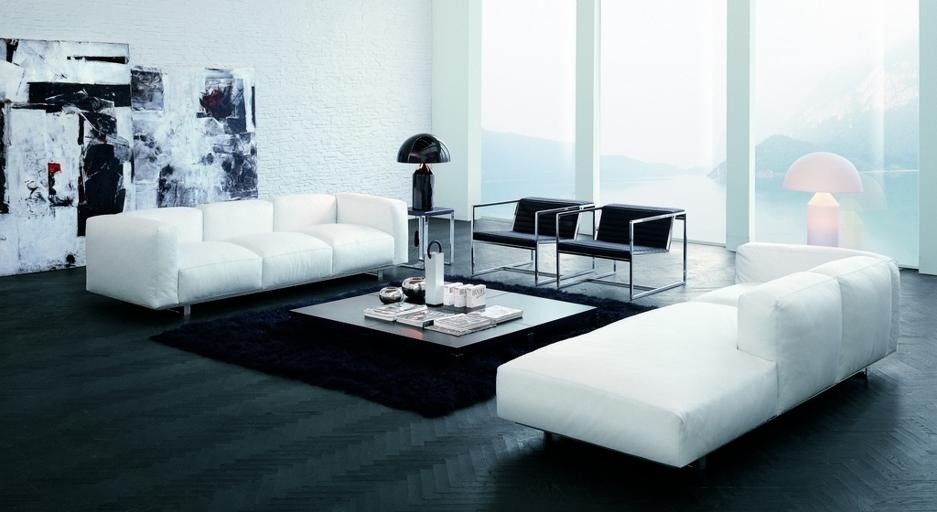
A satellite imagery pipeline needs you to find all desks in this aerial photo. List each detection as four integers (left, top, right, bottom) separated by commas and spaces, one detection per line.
401, 203, 456, 269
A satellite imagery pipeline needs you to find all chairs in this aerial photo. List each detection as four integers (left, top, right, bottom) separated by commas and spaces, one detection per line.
466, 194, 690, 305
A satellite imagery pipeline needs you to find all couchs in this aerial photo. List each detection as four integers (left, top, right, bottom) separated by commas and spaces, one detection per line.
491, 239, 901, 484
83, 186, 409, 316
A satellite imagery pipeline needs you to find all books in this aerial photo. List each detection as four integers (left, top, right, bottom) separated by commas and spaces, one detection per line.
424, 325, 496, 337
395, 309, 457, 328
433, 313, 492, 333
364, 301, 428, 322
466, 305, 523, 325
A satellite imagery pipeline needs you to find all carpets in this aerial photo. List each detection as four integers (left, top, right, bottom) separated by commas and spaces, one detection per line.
146, 273, 662, 419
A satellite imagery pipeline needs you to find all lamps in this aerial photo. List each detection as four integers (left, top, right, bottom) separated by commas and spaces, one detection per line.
783, 153, 865, 246
395, 133, 452, 211
